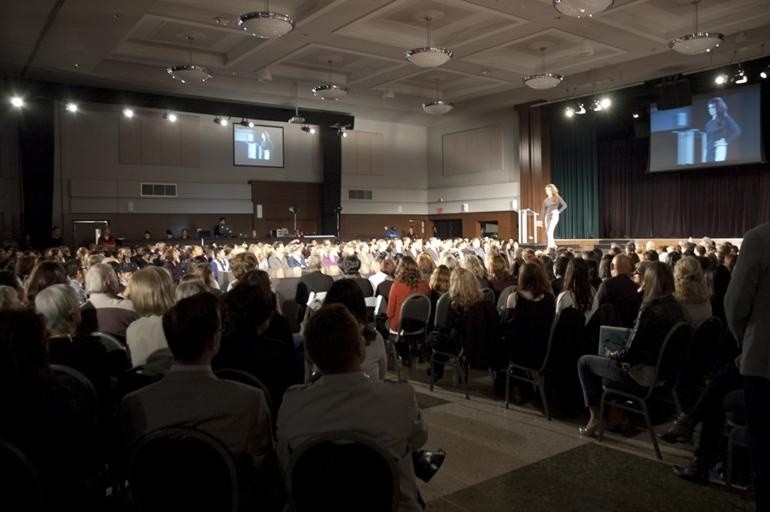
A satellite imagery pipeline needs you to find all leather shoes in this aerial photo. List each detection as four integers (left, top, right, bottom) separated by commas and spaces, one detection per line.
414, 449, 446, 483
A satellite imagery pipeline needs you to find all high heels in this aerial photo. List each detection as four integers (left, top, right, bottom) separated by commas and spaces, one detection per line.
577, 417, 604, 436
426, 367, 438, 383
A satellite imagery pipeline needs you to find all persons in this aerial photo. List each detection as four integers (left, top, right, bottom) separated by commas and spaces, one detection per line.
260, 131, 273, 159
701, 97, 741, 162
543, 184, 568, 255
3, 218, 765, 510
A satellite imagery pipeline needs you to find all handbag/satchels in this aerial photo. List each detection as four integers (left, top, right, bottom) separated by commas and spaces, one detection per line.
605, 402, 637, 436
435, 368, 461, 388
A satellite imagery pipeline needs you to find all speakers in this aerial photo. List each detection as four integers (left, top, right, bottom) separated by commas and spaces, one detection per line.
649, 74, 694, 111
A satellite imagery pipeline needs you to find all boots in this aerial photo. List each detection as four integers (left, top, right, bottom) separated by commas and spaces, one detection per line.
673, 455, 699, 483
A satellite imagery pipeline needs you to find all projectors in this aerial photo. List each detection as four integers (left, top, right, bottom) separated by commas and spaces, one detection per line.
288, 116, 305, 125
